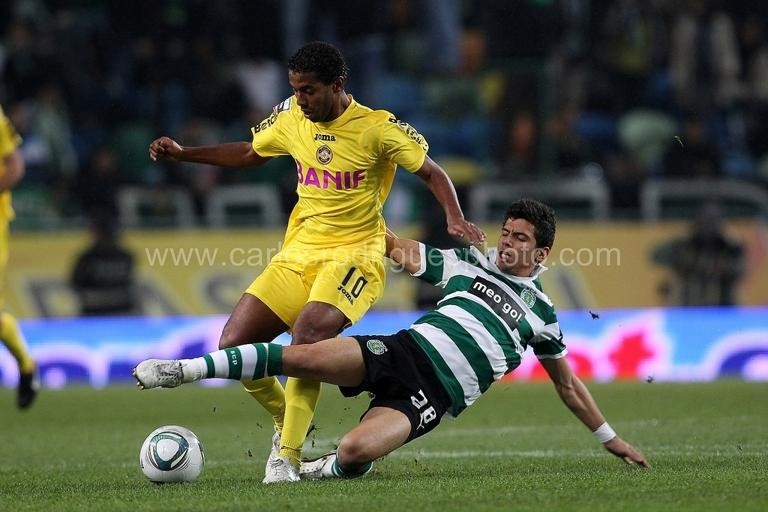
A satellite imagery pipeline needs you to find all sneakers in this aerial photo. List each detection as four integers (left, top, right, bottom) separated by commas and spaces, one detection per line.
262, 432, 301, 484
133, 359, 184, 390
17, 369, 39, 409
299, 451, 374, 480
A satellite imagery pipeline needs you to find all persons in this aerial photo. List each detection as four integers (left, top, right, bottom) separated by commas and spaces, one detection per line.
69, 216, 133, 313
148, 42, 487, 485
0, 2, 768, 221
661, 204, 745, 308
133, 198, 651, 479
0, 115, 40, 408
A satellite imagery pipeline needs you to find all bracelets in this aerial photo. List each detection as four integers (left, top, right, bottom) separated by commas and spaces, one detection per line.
592, 423, 618, 444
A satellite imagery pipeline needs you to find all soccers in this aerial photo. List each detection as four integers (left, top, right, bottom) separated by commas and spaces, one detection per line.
138, 425, 206, 488
